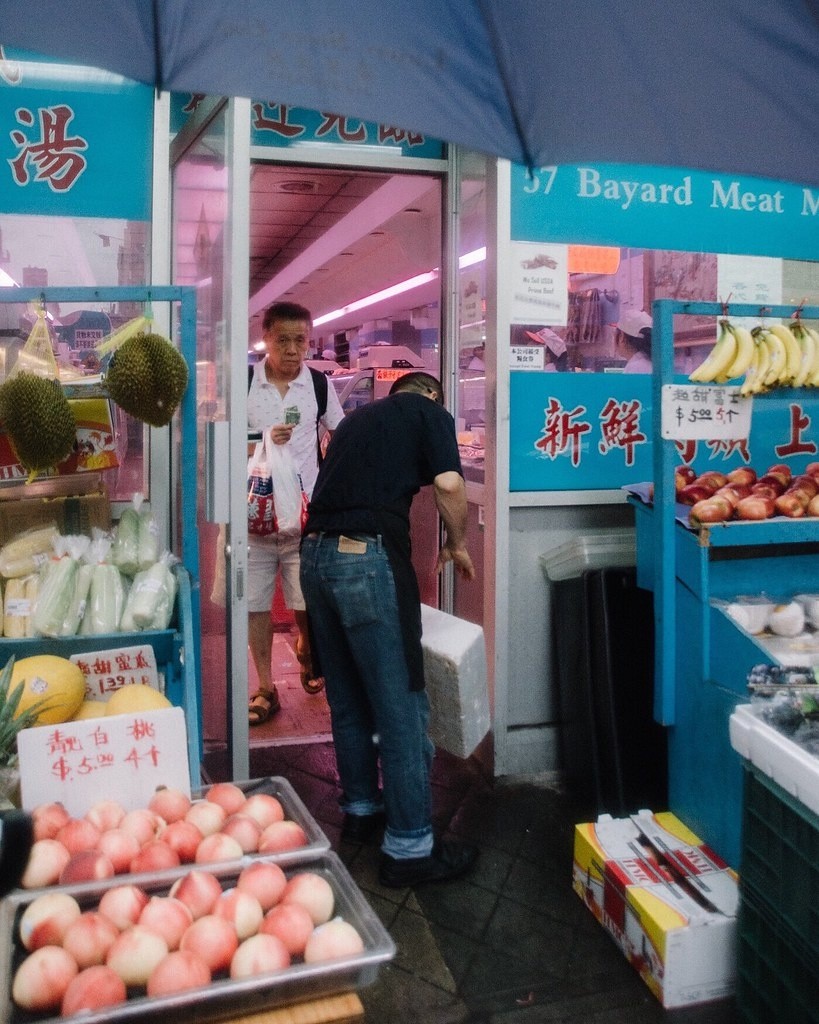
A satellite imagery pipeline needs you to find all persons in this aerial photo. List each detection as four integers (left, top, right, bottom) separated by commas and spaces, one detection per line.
211, 302, 345, 725
526, 328, 566, 372
321, 349, 336, 360
469, 341, 485, 371
613, 309, 653, 373
299, 373, 476, 886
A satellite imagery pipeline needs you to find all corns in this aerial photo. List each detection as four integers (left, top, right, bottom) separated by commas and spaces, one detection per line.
0, 509, 177, 638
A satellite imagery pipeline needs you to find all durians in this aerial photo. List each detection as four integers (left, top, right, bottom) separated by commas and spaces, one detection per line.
106, 331, 190, 428
0, 370, 76, 472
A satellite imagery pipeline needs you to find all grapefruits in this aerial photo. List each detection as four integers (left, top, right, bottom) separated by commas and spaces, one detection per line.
0, 655, 174, 730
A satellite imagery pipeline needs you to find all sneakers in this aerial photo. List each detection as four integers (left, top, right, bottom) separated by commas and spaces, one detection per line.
378, 838, 479, 887
341, 810, 389, 841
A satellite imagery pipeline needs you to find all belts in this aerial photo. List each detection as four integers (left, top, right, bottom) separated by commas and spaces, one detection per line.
307, 530, 377, 541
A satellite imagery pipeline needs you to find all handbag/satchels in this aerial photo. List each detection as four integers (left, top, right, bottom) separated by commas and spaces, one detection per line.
246, 426, 310, 542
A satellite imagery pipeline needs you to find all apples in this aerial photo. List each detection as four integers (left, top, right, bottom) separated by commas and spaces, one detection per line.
641, 461, 819, 526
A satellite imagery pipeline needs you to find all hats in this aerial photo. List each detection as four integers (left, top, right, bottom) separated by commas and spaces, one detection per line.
524, 327, 567, 358
320, 349, 337, 361
608, 310, 654, 339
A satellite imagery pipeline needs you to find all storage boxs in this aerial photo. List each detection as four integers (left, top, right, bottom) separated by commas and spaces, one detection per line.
540, 532, 668, 816
419, 602, 489, 759
0, 481, 112, 546
570, 810, 738, 1009
728, 702, 819, 1024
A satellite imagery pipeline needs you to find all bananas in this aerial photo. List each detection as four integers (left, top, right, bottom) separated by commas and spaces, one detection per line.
688, 318, 819, 398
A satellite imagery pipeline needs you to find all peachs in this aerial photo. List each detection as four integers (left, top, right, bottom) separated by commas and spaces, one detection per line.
19, 783, 309, 888
11, 860, 365, 1018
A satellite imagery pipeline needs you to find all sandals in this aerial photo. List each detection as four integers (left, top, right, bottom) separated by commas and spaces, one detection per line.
297, 655, 325, 694
248, 684, 280, 726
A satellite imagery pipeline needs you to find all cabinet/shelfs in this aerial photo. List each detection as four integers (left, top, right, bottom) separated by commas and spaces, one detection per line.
332, 327, 359, 368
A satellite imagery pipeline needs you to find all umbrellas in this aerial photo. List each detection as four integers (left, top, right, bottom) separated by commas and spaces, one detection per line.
0, 1, 819, 186
